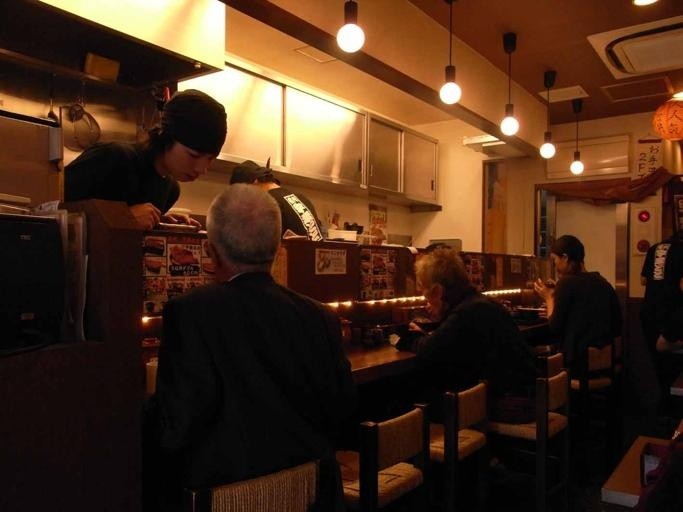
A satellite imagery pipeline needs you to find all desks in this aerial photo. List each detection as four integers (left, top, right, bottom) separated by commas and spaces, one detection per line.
599, 434, 682, 507
669, 371, 683, 394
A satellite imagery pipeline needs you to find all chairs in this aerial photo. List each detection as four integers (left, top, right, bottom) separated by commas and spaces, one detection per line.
571, 336, 621, 408
430, 381, 489, 511
333, 404, 431, 511
491, 347, 571, 504
192, 442, 327, 512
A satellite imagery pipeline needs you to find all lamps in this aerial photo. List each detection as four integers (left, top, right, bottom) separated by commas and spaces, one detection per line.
499, 29, 521, 137
539, 68, 558, 158
569, 96, 584, 177
336, 2, 366, 54
439, 1, 462, 106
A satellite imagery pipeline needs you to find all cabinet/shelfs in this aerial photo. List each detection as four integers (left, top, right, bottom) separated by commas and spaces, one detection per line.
391, 128, 439, 204
284, 86, 403, 195
179, 55, 285, 177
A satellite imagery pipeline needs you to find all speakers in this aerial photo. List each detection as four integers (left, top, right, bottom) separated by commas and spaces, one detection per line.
0, 214, 65, 357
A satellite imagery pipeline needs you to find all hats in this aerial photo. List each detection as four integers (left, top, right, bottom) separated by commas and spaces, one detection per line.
165, 90, 226, 154
553, 235, 583, 262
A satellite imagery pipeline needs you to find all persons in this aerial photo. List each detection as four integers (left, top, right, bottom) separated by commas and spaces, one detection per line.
229, 160, 326, 241
65, 90, 227, 234
388, 244, 543, 498
523, 234, 622, 381
639, 228, 682, 418
140, 182, 362, 512
